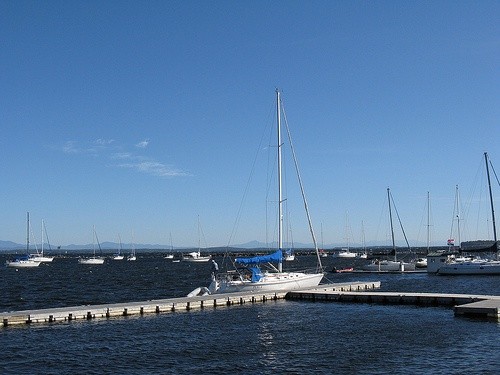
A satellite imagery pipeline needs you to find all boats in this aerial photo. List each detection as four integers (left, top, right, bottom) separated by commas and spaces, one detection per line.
332, 266, 353, 273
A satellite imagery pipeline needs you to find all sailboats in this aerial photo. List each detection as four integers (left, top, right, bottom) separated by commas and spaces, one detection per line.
128, 231, 137, 261
78, 223, 105, 265
318, 223, 328, 258
284, 211, 295, 261
165, 231, 174, 259
27, 216, 56, 262
114, 233, 125, 260
331, 209, 359, 257
362, 182, 464, 271
209, 89, 326, 294
180, 213, 211, 263
6, 211, 43, 267
436, 151, 500, 276
356, 219, 368, 259
